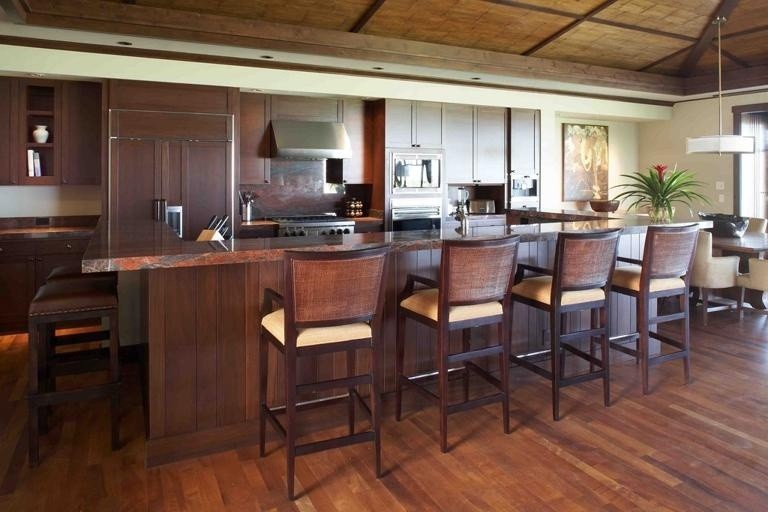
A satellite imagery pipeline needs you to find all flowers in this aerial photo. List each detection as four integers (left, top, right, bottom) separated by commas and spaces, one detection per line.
611, 163, 716, 220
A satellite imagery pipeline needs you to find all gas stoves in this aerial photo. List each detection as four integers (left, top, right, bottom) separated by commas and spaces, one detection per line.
273, 216, 355, 237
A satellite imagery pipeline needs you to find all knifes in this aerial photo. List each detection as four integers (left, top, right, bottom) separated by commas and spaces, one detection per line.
196, 215, 232, 241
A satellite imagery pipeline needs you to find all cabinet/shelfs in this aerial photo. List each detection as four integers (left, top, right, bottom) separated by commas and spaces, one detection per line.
508, 107, 540, 211
101, 78, 241, 241
1, 70, 101, 186
0, 231, 93, 333
445, 102, 507, 185
372, 96, 444, 149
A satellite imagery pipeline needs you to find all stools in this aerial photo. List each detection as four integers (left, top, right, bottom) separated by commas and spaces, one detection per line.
46, 266, 118, 391
28, 277, 122, 467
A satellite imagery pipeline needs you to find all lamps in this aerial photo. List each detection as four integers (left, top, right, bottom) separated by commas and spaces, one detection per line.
686, 17, 755, 154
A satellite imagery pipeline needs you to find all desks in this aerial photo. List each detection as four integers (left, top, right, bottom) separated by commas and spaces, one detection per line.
712, 235, 767, 311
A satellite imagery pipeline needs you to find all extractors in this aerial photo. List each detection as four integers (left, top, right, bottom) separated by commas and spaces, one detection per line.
269, 119, 353, 159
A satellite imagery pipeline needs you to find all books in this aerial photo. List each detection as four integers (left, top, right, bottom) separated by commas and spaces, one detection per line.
34, 153, 41, 177
27, 150, 34, 177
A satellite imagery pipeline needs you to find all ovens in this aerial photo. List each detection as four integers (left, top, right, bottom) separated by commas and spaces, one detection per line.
385, 228, 442, 244
384, 146, 447, 233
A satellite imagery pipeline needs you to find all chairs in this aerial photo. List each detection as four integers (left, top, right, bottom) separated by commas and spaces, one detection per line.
737, 257, 767, 319
590, 223, 699, 394
499, 227, 622, 421
691, 231, 740, 325
746, 217, 768, 237
394, 234, 521, 453
258, 245, 393, 500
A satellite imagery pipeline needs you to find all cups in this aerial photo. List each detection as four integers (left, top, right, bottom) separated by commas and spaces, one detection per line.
242, 204, 252, 222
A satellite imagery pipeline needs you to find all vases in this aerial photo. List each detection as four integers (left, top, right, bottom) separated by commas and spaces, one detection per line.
647, 207, 675, 222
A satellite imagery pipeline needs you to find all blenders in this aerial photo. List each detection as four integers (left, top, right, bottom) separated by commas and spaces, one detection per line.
456, 186, 469, 215
